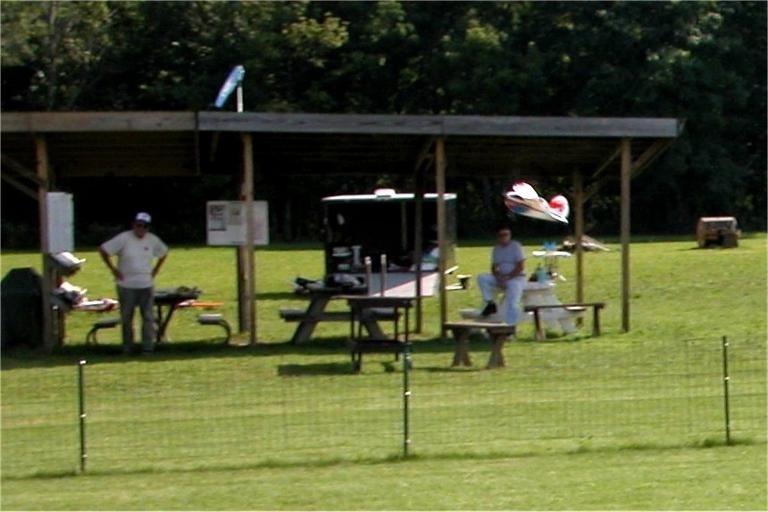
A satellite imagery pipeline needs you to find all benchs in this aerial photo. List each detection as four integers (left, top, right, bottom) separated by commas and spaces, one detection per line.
84, 312, 233, 353
441, 300, 607, 376
279, 309, 407, 354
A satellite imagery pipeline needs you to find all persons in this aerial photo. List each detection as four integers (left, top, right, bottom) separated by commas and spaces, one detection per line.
476, 226, 531, 342
98, 211, 171, 358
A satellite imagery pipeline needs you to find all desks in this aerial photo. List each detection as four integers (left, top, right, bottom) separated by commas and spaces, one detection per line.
521, 280, 584, 335
292, 276, 417, 344
151, 286, 196, 355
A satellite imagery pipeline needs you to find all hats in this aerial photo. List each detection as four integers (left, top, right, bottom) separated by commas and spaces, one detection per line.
135, 213, 152, 223
496, 223, 511, 231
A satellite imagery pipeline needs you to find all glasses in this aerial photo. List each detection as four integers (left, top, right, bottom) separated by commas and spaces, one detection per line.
136, 224, 148, 228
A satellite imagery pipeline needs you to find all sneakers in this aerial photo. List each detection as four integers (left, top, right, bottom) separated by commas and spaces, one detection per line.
481, 303, 496, 316
